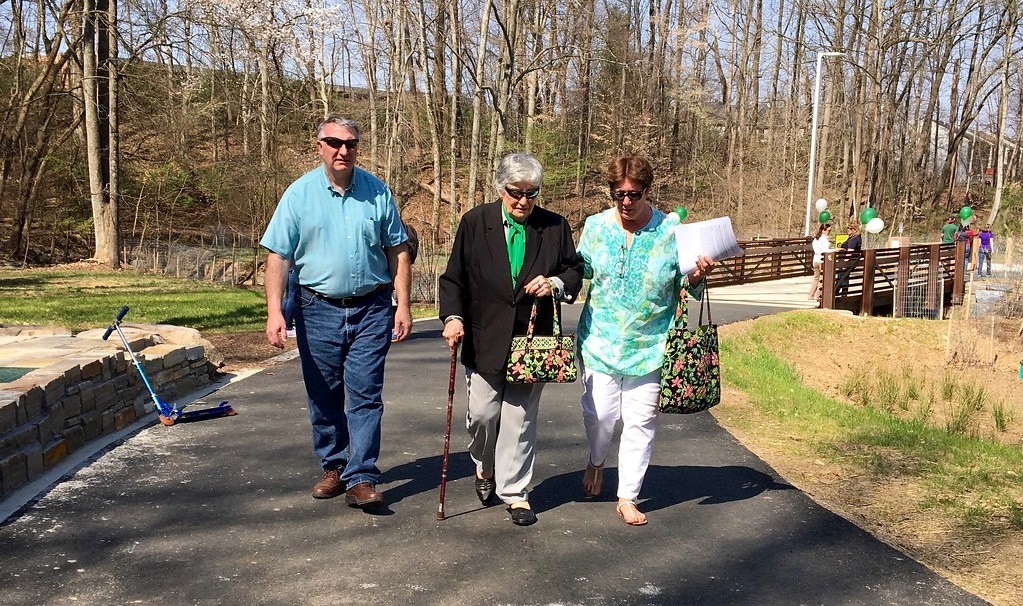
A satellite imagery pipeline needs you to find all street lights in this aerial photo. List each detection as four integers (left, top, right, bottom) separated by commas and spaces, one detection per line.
804, 52, 846, 237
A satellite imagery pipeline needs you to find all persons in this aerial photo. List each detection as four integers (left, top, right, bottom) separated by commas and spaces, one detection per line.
388, 219, 404, 343
808, 223, 833, 302
977, 224, 994, 277
439, 153, 585, 524
259, 117, 413, 506
277, 260, 297, 338
575, 157, 716, 525
941, 216, 974, 274
835, 220, 862, 298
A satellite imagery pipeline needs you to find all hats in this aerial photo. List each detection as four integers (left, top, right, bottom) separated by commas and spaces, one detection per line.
983, 225, 991, 231
947, 216, 955, 223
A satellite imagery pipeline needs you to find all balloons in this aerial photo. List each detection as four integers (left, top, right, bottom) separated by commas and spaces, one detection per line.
860, 208, 877, 224
667, 206, 687, 225
816, 198, 827, 212
866, 218, 884, 233
960, 206, 972, 220
961, 216, 971, 227
819, 211, 830, 223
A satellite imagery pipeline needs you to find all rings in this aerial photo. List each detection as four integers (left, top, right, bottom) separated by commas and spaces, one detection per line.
537, 283, 541, 287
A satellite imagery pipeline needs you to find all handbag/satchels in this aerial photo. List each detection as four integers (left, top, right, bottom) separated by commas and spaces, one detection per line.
507, 278, 577, 383
658, 276, 721, 414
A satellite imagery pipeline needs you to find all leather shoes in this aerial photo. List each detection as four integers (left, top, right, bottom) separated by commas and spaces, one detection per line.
346, 482, 384, 505
475, 464, 495, 503
510, 502, 536, 525
313, 470, 344, 498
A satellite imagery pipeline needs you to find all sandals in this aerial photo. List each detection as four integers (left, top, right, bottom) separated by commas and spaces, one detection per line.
616, 500, 648, 525
583, 459, 604, 496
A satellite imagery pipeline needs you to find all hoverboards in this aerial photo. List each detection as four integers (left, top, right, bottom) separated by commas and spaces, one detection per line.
102, 306, 237, 427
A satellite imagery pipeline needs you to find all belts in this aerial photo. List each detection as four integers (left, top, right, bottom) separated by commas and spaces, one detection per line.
323, 285, 386, 307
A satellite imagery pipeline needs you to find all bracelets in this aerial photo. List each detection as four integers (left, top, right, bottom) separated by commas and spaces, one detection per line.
444, 317, 462, 324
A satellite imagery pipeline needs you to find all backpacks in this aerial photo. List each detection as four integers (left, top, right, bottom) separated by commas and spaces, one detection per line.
404, 224, 419, 264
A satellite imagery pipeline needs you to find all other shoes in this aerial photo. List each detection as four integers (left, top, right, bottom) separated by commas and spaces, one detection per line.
286, 326, 297, 338
391, 330, 398, 343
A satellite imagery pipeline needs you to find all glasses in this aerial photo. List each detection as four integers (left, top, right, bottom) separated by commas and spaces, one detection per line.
611, 188, 647, 201
320, 137, 359, 150
505, 186, 539, 199
847, 227, 854, 230
614, 245, 628, 278
